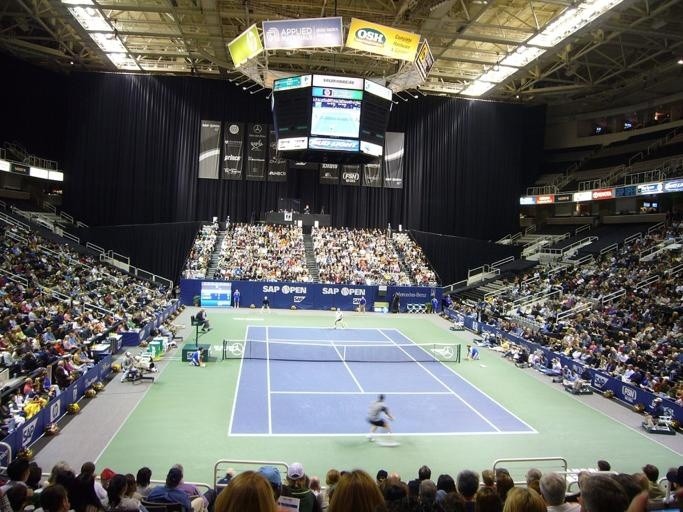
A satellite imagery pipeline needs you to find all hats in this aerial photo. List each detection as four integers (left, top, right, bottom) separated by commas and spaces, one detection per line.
286, 462, 305, 481
101, 468, 117, 480
377, 470, 387, 482
256, 465, 282, 487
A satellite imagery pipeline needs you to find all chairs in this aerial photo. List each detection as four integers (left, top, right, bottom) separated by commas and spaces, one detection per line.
138, 497, 191, 512
183, 220, 438, 288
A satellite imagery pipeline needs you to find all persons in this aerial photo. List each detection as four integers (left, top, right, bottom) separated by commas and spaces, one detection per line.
366, 393, 398, 446
233, 289, 240, 309
641, 397, 664, 428
260, 295, 270, 312
430, 296, 437, 314
187, 346, 204, 366
358, 296, 366, 314
1, 220, 182, 443
392, 293, 400, 313
180, 204, 438, 289
1, 454, 682, 510
439, 205, 682, 409
195, 308, 212, 332
333, 306, 346, 330
